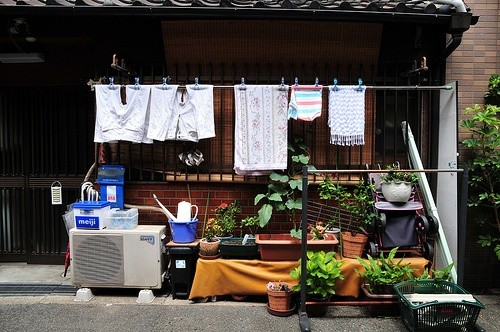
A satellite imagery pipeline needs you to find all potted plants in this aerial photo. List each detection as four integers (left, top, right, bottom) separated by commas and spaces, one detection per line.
254, 143, 338, 261
379, 163, 420, 202
266, 281, 296, 316
352, 246, 420, 315
198, 234, 221, 260
319, 174, 376, 258
290, 249, 345, 316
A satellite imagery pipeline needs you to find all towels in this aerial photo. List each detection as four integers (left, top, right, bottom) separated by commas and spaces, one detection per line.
230, 83, 287, 176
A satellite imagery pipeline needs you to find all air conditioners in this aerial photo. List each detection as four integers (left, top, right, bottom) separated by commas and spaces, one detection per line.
68, 225, 166, 289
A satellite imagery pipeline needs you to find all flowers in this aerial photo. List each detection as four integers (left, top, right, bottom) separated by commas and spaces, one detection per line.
206, 200, 242, 236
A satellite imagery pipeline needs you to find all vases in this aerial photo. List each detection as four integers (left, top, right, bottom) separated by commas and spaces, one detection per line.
215, 234, 233, 240
220, 238, 258, 260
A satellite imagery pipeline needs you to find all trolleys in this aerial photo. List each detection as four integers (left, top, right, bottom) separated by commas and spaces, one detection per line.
365, 163, 439, 260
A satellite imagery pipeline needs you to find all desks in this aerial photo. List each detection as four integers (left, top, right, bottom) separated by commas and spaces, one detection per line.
188, 257, 431, 300
166, 240, 199, 300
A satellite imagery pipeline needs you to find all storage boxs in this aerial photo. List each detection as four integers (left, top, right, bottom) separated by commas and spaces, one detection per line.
71, 201, 139, 229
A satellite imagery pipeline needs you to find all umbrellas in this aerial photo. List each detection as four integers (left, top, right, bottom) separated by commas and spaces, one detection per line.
62, 182, 99, 277
